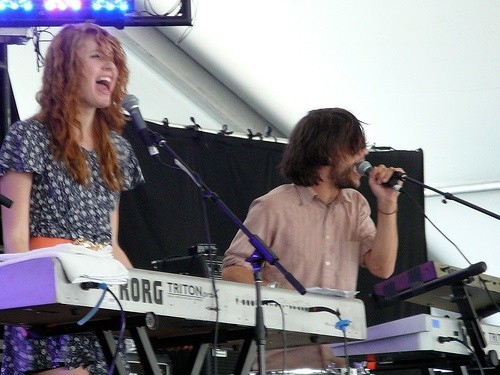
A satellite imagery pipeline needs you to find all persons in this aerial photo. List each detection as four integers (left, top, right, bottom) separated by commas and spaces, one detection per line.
0, 23, 145, 375
221, 107, 406, 375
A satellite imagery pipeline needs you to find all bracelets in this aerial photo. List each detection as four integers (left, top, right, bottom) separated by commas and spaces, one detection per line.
377, 209, 398, 215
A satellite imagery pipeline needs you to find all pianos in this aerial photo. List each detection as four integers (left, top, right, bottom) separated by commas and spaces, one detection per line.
0, 244, 369, 375
331, 259, 500, 375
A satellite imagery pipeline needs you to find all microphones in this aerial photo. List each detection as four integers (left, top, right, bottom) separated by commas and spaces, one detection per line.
358, 160, 404, 192
123, 94, 160, 159
